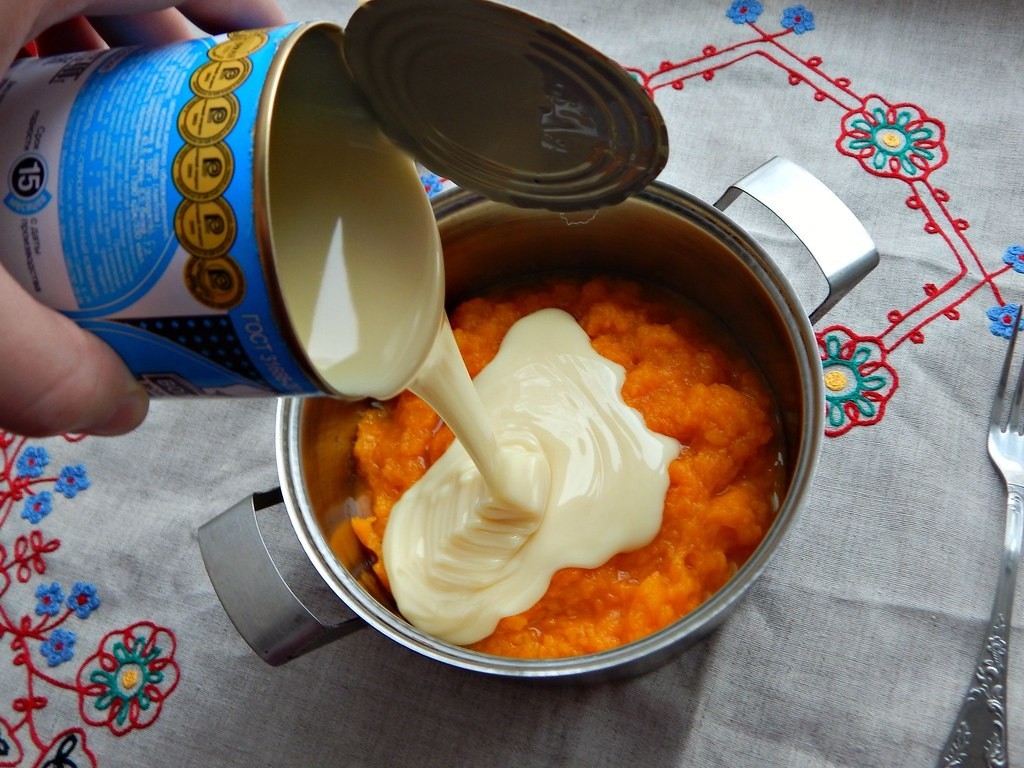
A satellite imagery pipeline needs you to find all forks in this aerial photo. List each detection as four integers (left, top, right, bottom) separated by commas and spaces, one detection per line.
934, 306, 1023, 768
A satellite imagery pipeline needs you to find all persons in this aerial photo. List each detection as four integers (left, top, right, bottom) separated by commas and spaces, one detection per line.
0, 0, 302, 438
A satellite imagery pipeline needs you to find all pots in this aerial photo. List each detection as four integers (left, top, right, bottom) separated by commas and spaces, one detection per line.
190, 156, 881, 690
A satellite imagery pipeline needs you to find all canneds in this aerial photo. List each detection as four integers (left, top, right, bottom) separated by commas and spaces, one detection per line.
0, 0, 670, 406
